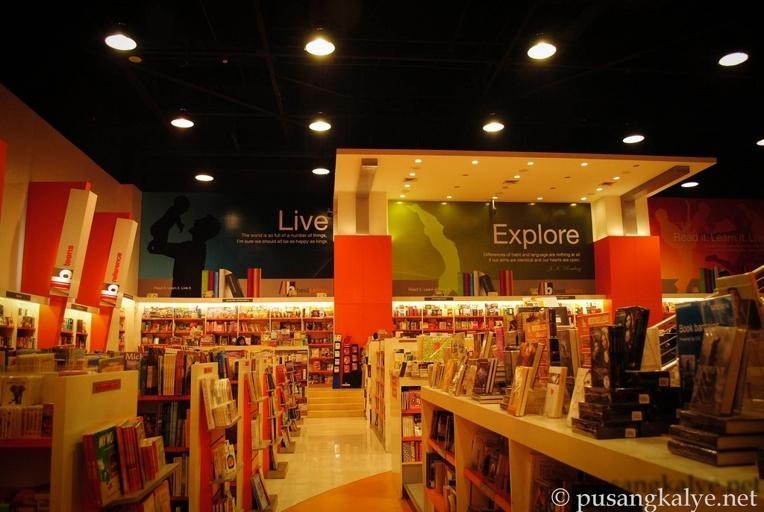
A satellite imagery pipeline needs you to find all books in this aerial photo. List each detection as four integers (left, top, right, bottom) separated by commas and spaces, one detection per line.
0, 273, 764, 512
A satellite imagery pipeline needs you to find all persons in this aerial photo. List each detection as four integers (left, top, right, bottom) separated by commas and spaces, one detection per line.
236, 336, 248, 345
